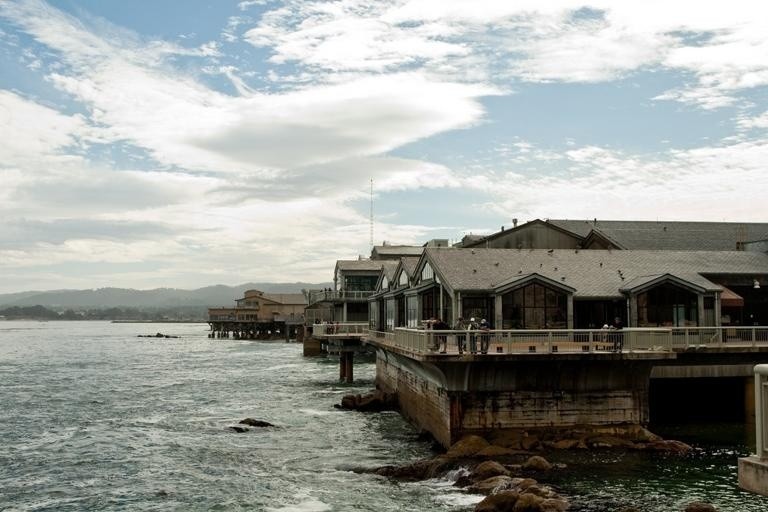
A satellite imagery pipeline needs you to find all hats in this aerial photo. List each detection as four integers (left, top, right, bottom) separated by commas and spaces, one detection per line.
458, 316, 488, 324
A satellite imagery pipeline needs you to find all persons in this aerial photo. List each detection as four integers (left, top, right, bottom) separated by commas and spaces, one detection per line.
339, 287, 343, 298
468, 317, 480, 354
320, 287, 331, 292
326, 321, 340, 334
611, 317, 624, 352
435, 319, 448, 353
455, 317, 467, 354
478, 319, 491, 354
